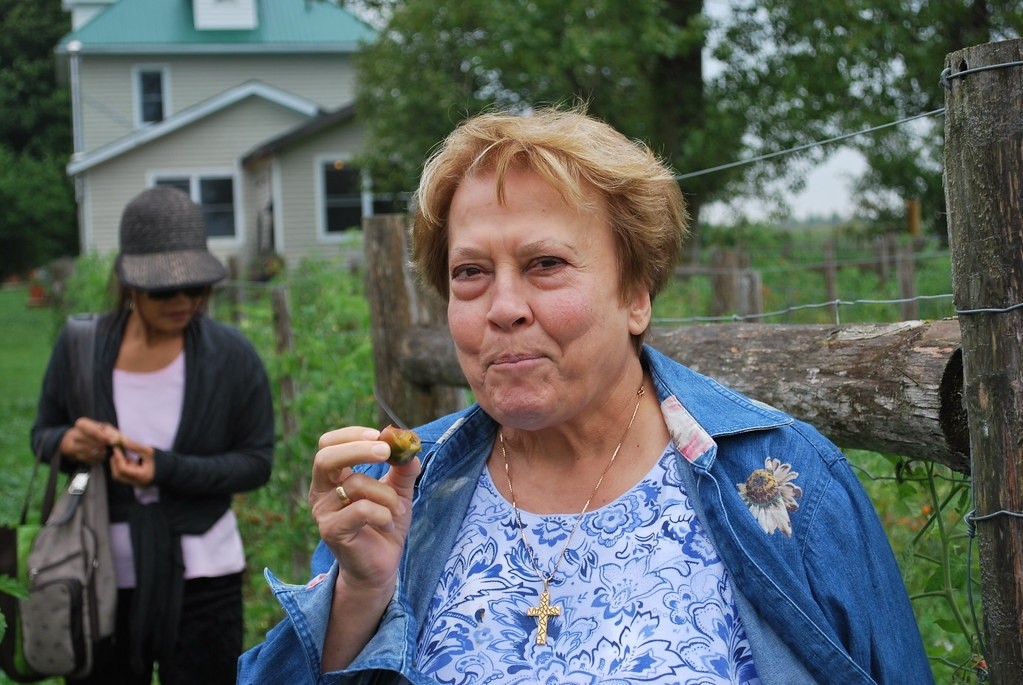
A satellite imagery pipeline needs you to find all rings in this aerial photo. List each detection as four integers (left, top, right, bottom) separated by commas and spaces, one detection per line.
335, 482, 352, 505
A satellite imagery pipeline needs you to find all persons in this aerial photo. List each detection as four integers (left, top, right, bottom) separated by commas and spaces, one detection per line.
30, 184, 276, 685
233, 111, 939, 685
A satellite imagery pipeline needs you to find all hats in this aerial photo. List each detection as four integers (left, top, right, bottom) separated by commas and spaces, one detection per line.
117, 184, 225, 292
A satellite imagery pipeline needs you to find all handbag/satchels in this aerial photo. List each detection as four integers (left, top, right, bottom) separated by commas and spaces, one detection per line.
0, 424, 77, 685
20, 460, 118, 682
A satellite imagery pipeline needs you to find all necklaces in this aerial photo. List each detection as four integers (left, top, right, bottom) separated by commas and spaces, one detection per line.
498, 384, 645, 646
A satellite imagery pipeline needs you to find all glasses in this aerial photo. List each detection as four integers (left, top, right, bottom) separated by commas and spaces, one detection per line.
147, 283, 207, 300
